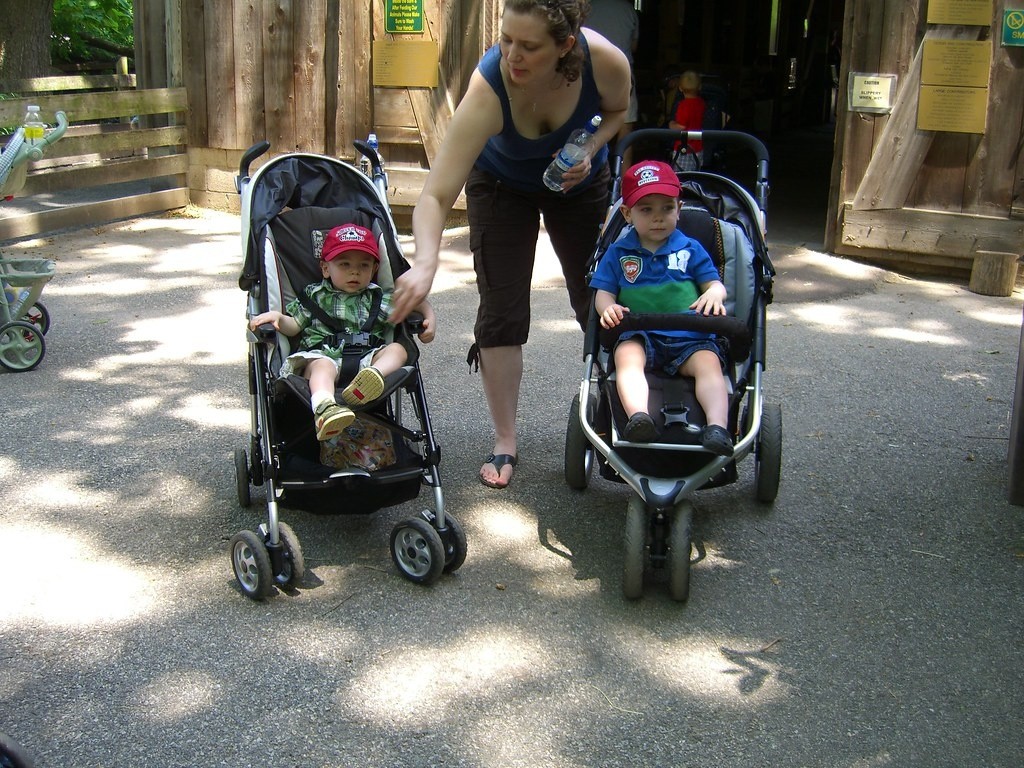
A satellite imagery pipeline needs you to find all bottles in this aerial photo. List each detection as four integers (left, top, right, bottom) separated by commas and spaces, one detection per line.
25, 106, 44, 146
359, 134, 385, 181
543, 115, 602, 192
10, 287, 32, 320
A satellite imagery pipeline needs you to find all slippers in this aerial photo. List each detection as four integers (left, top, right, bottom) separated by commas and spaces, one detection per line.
479, 445, 519, 488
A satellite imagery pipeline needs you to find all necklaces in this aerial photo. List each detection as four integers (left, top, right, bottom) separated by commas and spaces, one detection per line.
520, 87, 539, 115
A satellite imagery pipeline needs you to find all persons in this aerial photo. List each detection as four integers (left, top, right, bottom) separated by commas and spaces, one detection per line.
590, 161, 734, 457
669, 69, 707, 172
390, 0, 631, 489
250, 224, 435, 440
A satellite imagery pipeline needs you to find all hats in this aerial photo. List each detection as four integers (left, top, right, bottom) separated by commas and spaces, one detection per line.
622, 160, 683, 208
321, 224, 380, 261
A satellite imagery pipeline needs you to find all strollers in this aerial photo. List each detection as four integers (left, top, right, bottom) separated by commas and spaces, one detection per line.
230, 139, 469, 601
564, 128, 783, 604
1, 110, 66, 373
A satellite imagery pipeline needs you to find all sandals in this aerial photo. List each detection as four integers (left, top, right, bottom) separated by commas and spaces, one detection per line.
623, 412, 661, 444
697, 424, 734, 458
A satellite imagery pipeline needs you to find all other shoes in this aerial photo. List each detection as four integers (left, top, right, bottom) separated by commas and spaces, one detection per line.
314, 399, 355, 440
341, 366, 385, 407
